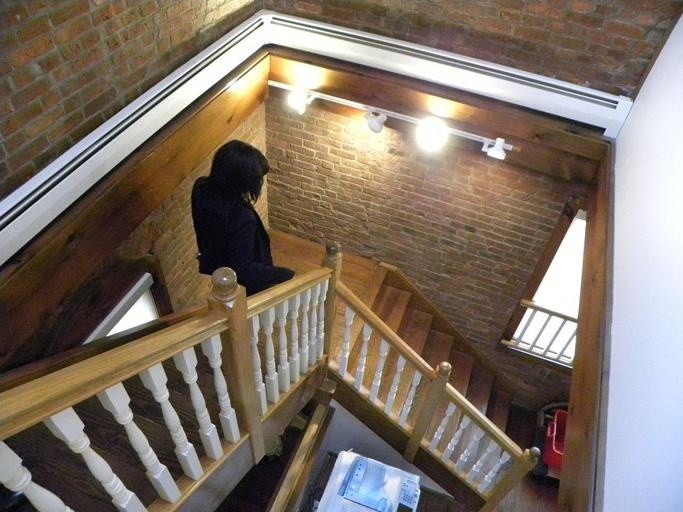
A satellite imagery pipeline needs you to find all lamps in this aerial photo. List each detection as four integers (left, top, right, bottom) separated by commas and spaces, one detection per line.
481, 135, 508, 162
362, 105, 388, 135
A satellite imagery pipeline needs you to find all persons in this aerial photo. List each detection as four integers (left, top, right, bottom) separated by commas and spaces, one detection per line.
191, 140, 295, 298
